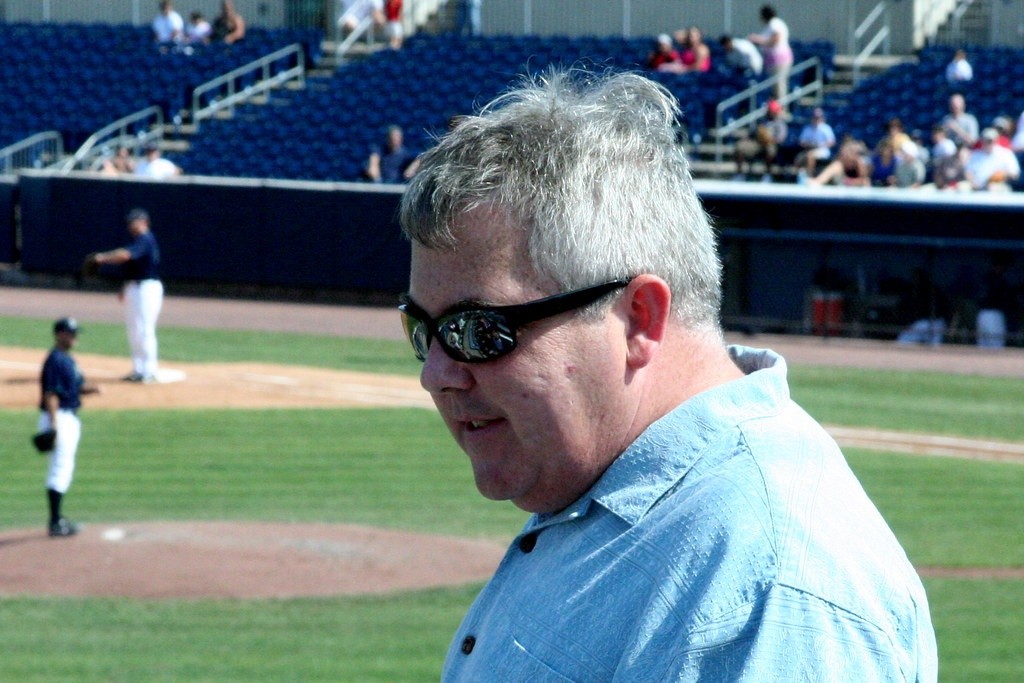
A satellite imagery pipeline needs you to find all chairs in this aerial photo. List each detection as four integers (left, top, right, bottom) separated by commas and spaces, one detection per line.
0, 23, 1023, 193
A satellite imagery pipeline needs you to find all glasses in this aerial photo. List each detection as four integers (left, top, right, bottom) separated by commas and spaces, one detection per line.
397, 274, 640, 364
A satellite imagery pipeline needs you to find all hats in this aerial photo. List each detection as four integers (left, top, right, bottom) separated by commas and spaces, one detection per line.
129, 209, 149, 223
54, 315, 80, 332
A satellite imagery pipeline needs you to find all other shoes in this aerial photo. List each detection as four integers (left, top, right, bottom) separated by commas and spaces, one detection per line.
48, 520, 85, 534
124, 372, 154, 383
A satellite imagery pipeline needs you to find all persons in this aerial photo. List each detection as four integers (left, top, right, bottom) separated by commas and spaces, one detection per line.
719, 37, 763, 80
647, 26, 710, 73
944, 51, 971, 85
88, 210, 163, 381
370, 126, 420, 185
396, 68, 939, 683
38, 317, 99, 536
896, 308, 1007, 347
150, 0, 245, 48
342, 0, 405, 52
89, 143, 184, 177
747, 5, 793, 98
736, 94, 1024, 192
